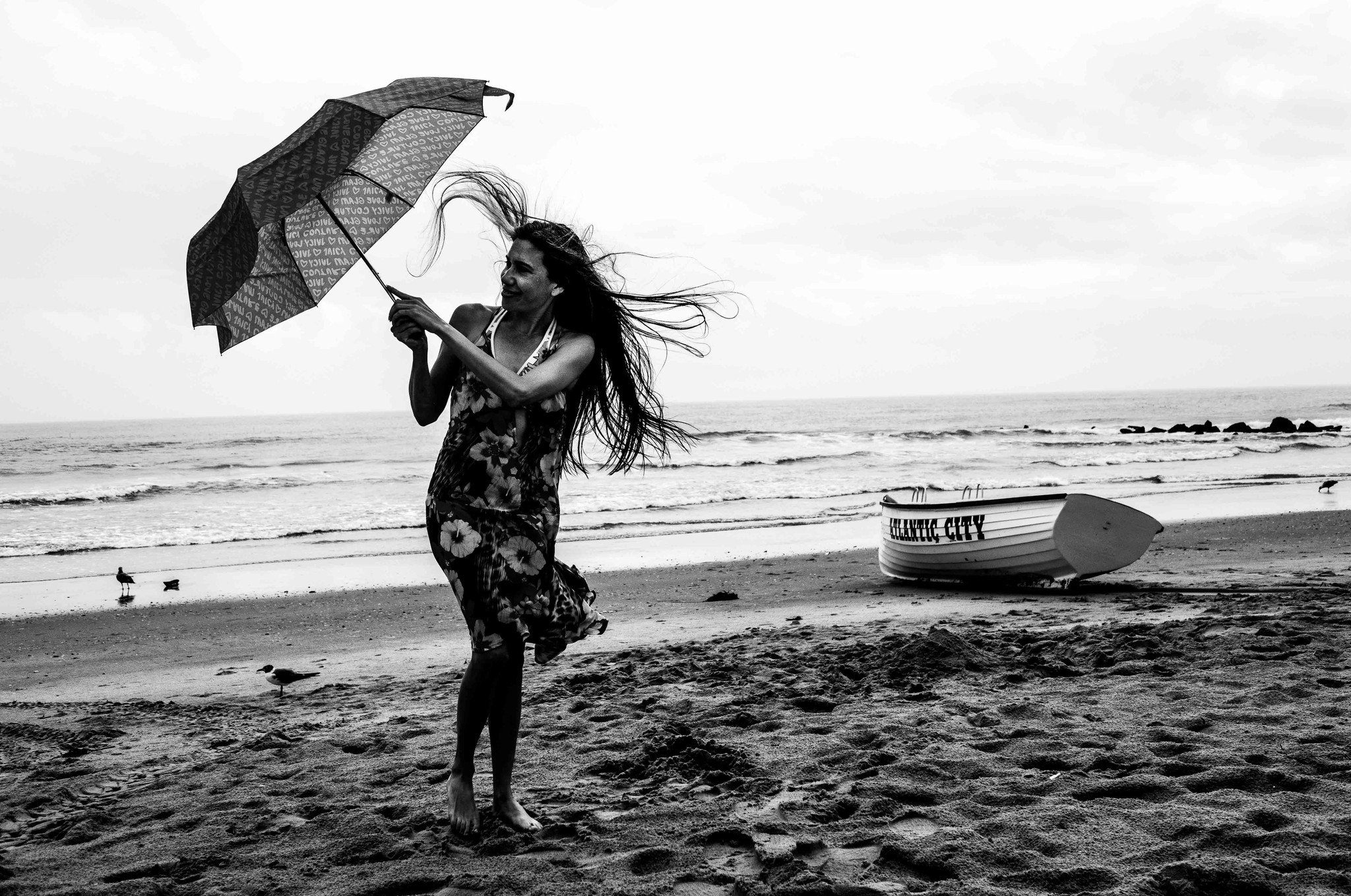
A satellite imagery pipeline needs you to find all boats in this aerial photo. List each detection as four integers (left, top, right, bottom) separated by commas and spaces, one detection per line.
878, 484, 1165, 590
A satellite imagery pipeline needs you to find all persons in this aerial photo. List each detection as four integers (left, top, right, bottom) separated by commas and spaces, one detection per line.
384, 219, 699, 837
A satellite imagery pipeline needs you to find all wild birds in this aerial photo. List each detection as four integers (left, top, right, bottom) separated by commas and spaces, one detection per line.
115, 567, 136, 590
1317, 479, 1339, 494
255, 664, 322, 699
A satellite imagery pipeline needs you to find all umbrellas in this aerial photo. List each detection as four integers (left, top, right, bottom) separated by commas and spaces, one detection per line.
184, 76, 518, 337
